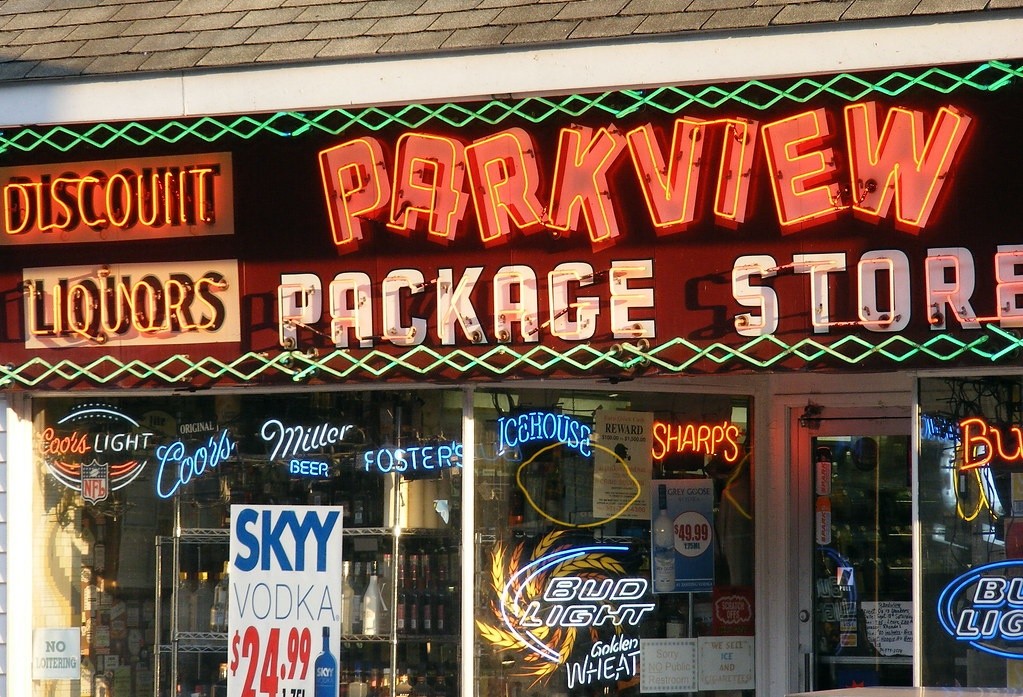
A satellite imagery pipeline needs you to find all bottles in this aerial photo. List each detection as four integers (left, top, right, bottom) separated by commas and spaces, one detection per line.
314, 627, 337, 697
340, 661, 462, 697
653, 484, 675, 593
178, 536, 462, 636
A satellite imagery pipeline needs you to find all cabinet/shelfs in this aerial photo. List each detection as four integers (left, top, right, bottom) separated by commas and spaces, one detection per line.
169, 405, 462, 697
